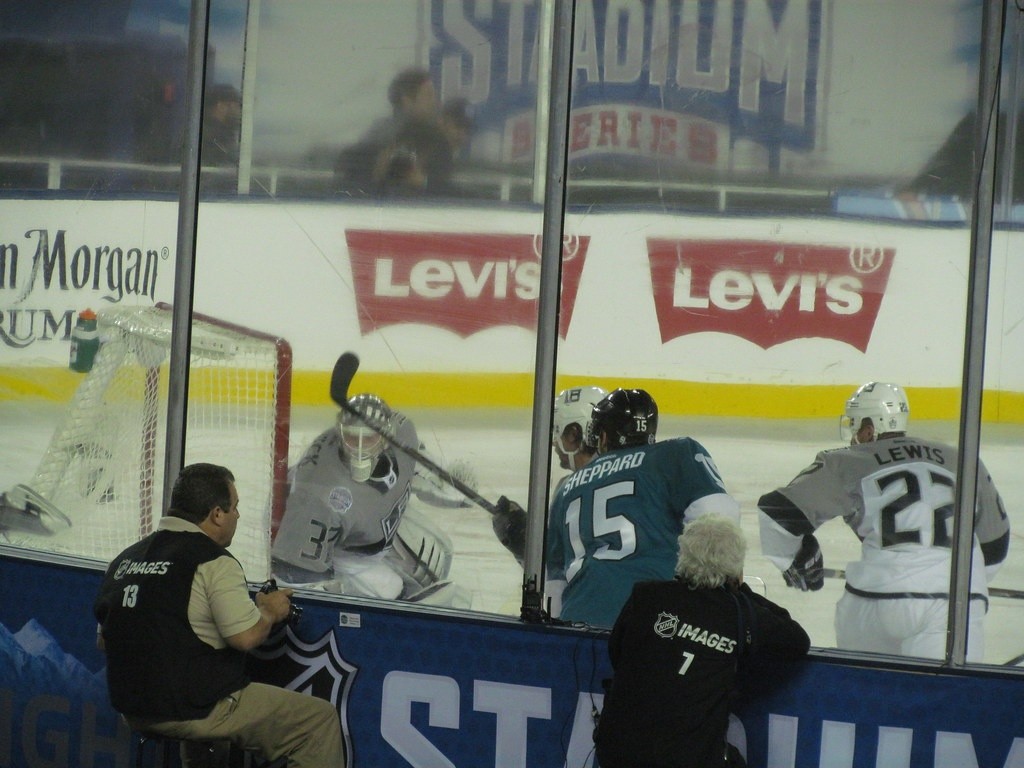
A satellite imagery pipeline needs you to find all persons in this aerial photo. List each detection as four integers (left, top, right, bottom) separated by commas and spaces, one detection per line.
757, 379, 1012, 664
490, 383, 744, 628
131, 69, 482, 201
270, 393, 479, 607
91, 463, 346, 767
590, 510, 812, 768
1, 480, 72, 539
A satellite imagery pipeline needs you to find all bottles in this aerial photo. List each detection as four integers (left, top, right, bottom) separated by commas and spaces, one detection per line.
69, 307, 100, 373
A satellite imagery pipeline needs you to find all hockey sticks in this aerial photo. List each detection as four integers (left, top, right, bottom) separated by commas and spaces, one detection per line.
329, 352, 496, 511
821, 567, 1024, 600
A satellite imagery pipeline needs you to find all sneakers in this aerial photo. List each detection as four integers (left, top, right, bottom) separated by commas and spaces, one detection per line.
0, 484, 72, 537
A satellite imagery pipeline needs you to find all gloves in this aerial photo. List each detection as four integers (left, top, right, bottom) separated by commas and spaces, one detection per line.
493, 495, 527, 559
784, 535, 824, 592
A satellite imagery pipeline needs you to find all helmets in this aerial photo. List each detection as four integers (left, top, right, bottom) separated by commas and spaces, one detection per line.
591, 388, 658, 451
336, 393, 394, 481
552, 385, 606, 449
846, 381, 909, 441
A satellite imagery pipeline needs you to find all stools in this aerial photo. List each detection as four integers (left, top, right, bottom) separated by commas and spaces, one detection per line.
134, 732, 249, 768
